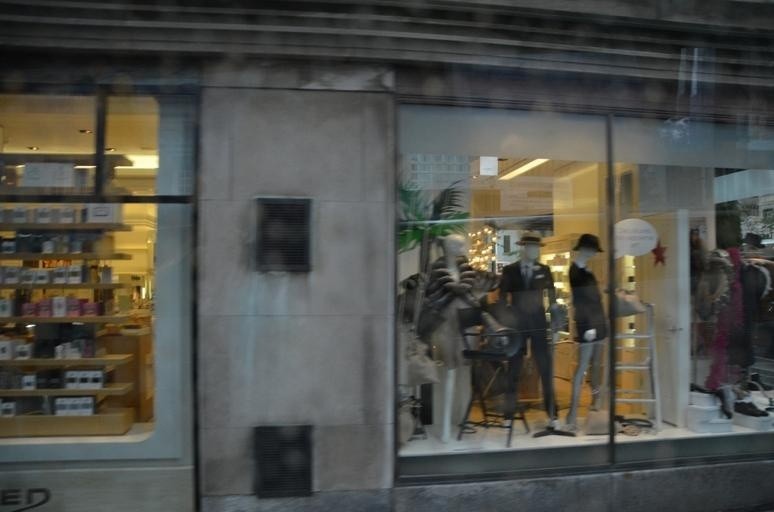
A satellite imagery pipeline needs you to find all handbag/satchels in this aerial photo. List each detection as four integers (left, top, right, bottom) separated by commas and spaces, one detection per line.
615, 287, 647, 318
407, 344, 445, 386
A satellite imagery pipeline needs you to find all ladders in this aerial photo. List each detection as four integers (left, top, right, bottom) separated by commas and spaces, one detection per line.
607, 304, 661, 431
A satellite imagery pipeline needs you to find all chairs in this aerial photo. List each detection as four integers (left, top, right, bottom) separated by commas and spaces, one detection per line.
455, 303, 530, 448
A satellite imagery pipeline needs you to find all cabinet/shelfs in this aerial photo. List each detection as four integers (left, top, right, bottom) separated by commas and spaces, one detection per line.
546, 231, 574, 337
0, 152, 154, 438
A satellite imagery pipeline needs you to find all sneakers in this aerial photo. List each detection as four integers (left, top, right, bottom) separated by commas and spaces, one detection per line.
504, 420, 512, 431
549, 419, 561, 430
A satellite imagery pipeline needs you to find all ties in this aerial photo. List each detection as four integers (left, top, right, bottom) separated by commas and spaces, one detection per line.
524, 264, 529, 288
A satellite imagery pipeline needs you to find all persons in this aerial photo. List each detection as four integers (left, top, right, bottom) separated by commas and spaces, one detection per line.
696, 232, 767, 389
425, 232, 607, 442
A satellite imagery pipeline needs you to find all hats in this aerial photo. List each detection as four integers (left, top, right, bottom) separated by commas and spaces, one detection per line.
515, 233, 546, 246
573, 234, 605, 252
741, 233, 766, 248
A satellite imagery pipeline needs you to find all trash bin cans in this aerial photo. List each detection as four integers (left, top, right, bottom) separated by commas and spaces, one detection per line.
255, 426, 312, 498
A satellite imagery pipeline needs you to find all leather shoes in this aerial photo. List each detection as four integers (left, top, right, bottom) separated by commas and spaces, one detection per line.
734, 402, 769, 416
